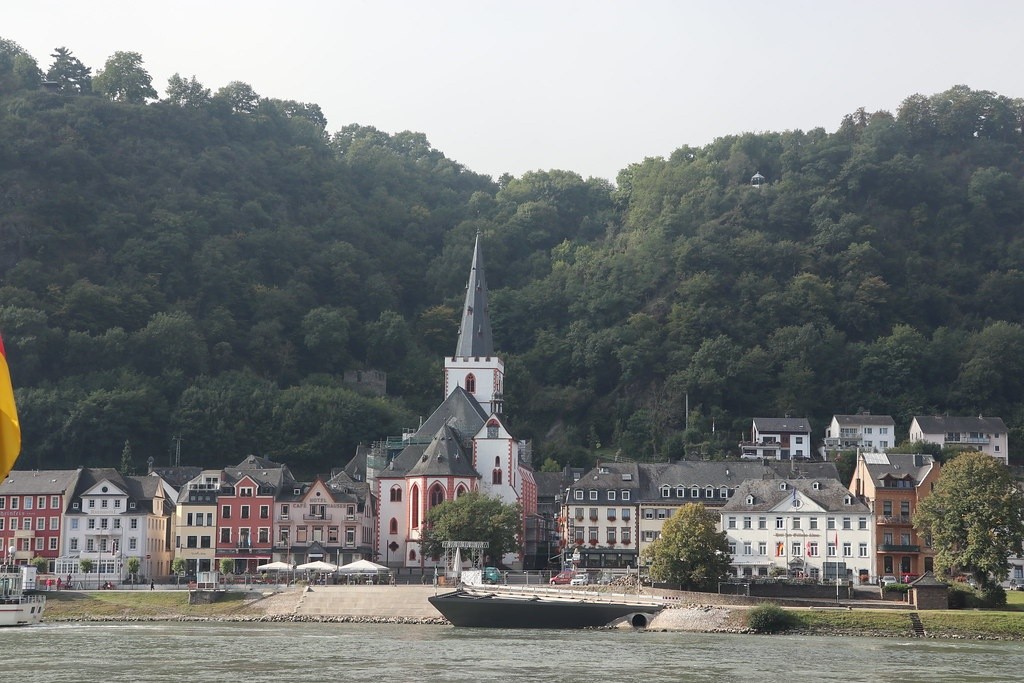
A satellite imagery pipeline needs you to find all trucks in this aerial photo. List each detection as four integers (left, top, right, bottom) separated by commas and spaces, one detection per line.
481, 567, 503, 585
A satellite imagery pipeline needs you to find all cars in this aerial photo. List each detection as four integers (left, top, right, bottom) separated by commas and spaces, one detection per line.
966, 578, 979, 589
1010, 578, 1024, 591
881, 576, 897, 587
570, 574, 589, 586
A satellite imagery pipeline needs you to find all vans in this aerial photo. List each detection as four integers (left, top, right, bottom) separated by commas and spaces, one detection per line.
550, 570, 577, 585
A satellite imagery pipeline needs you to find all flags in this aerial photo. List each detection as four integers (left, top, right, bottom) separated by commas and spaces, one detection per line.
777, 541, 783, 556
836, 531, 837, 550
0, 335, 21, 487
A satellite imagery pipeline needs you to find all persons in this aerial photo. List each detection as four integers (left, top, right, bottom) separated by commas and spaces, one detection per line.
503, 571, 509, 586
151, 578, 154, 590
45, 573, 73, 591
102, 580, 115, 590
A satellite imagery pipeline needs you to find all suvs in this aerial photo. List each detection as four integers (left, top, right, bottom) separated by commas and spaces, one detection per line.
774, 575, 790, 581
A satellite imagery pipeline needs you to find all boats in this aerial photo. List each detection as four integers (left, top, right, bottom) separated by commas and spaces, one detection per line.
0, 544, 46, 628
428, 582, 669, 631
